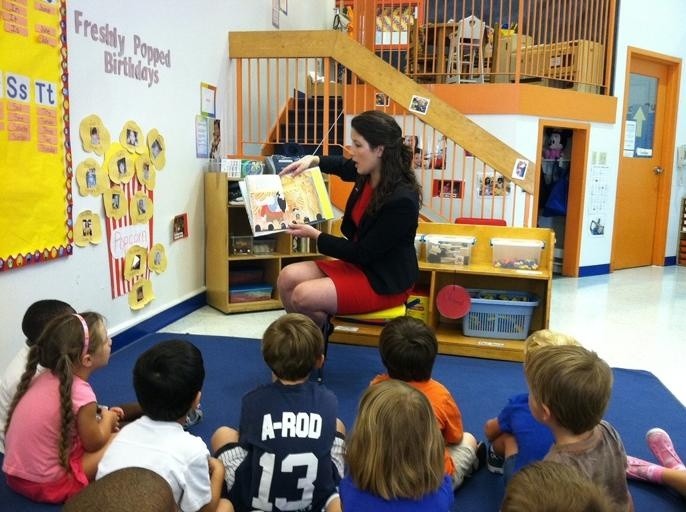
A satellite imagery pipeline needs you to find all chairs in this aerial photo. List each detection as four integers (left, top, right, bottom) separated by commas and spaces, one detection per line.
447, 14, 486, 85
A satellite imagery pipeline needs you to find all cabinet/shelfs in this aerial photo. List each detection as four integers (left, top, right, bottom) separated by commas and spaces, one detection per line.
406, 23, 459, 84
510, 39, 605, 94
326, 219, 557, 361
204, 170, 332, 361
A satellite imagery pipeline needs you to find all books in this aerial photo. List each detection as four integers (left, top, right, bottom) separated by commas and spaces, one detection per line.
238, 164, 336, 239
293, 234, 313, 255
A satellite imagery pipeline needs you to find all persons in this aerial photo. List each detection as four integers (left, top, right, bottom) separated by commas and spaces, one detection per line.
499, 460, 618, 511
137, 198, 146, 215
274, 108, 421, 332
481, 176, 505, 196
117, 159, 127, 174
2, 298, 203, 465
486, 328, 581, 478
324, 380, 455, 510
112, 194, 119, 209
127, 130, 137, 145
211, 311, 346, 511
370, 316, 479, 493
96, 339, 234, 510
443, 183, 451, 198
625, 426, 686, 500
90, 127, 99, 145
525, 344, 632, 510
82, 220, 92, 237
209, 311, 346, 511
62, 467, 181, 509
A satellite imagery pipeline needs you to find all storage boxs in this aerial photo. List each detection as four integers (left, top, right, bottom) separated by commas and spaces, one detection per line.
425, 234, 477, 266
488, 237, 545, 271
414, 233, 425, 262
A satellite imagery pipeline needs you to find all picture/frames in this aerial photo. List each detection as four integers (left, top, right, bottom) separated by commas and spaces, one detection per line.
271, 9, 280, 28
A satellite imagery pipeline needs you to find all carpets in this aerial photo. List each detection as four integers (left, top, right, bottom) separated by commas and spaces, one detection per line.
0, 330, 686, 512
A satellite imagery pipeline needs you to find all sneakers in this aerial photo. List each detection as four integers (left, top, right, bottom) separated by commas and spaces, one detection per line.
185, 405, 201, 431
625, 456, 665, 484
476, 440, 503, 476
646, 429, 684, 467
